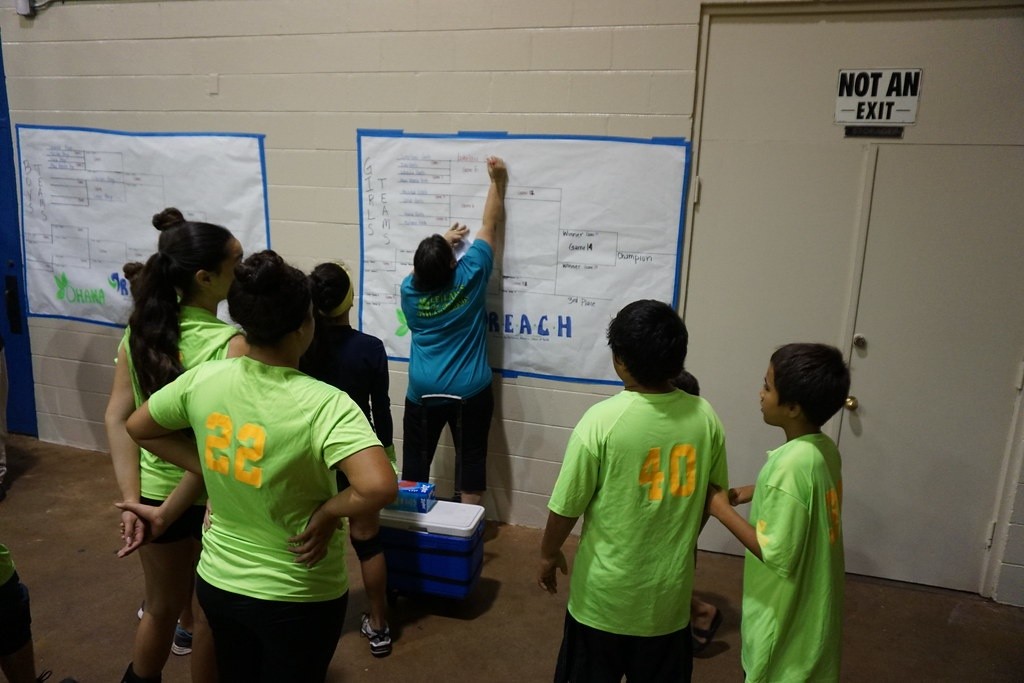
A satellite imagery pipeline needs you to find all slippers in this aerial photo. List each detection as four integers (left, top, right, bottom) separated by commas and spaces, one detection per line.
690, 598, 723, 654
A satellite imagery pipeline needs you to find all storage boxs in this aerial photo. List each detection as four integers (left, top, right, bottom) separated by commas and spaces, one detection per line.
379, 500, 487, 619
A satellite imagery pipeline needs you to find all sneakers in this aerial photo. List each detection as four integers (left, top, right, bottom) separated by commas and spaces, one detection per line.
360, 609, 392, 658
137, 600, 144, 620
171, 624, 192, 656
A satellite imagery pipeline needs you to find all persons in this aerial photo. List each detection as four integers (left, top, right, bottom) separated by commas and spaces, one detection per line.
665, 371, 724, 653
125, 247, 401, 683
703, 341, 852, 682
0, 542, 75, 682
297, 260, 397, 658
103, 205, 243, 682
397, 153, 510, 506
535, 299, 728, 682
